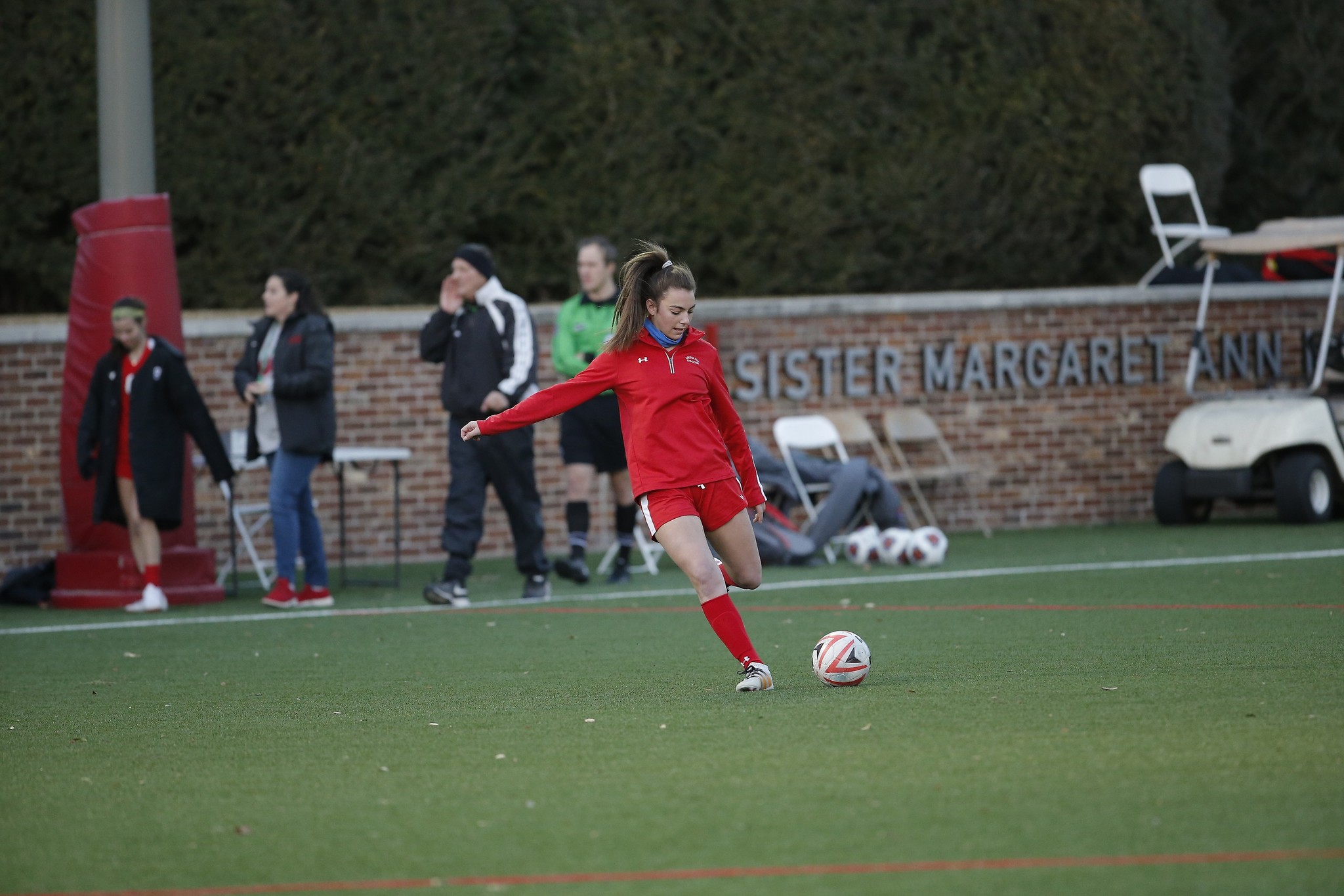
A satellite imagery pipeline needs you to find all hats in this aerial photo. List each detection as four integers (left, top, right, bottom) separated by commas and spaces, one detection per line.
454, 243, 494, 281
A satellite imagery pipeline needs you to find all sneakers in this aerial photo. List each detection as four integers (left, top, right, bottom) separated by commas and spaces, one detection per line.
261, 577, 298, 608
142, 583, 163, 612
297, 583, 334, 608
523, 574, 547, 601
423, 579, 470, 607
607, 571, 632, 590
554, 559, 589, 586
735, 662, 774, 693
124, 592, 168, 612
712, 557, 730, 594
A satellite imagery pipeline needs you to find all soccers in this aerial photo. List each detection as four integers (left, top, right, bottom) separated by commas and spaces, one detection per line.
844, 525, 877, 564
810, 631, 870, 686
875, 526, 912, 564
906, 525, 948, 567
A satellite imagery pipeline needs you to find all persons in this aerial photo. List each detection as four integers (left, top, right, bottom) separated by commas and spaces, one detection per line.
234, 266, 337, 609
551, 237, 640, 585
459, 238, 776, 693
73, 297, 235, 615
418, 242, 557, 605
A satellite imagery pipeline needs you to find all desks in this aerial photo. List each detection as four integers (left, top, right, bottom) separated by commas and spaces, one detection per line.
189, 443, 417, 596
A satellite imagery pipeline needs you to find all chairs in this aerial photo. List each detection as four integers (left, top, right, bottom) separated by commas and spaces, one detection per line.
774, 413, 888, 563
212, 425, 309, 589
820, 411, 921, 536
1133, 162, 1234, 288
881, 403, 996, 540
